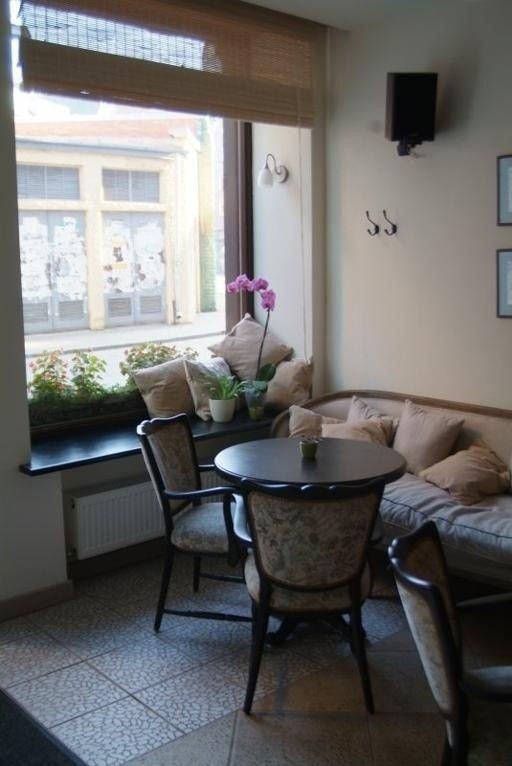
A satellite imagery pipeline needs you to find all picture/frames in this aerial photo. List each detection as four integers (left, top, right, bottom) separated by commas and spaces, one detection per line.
497, 155, 511, 226
497, 249, 512, 318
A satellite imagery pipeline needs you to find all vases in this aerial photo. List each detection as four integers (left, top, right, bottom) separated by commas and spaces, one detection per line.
248, 406, 265, 422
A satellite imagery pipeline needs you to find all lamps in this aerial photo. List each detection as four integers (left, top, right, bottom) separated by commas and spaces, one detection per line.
257, 154, 288, 186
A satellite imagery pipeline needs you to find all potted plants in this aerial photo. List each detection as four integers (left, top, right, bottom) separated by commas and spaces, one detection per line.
296, 434, 322, 458
200, 373, 246, 422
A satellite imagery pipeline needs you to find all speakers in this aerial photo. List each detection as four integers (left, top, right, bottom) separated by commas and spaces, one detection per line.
384, 71, 438, 145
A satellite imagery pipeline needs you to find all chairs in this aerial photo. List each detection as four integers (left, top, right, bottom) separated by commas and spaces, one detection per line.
387, 519, 511, 765
136, 413, 257, 641
237, 474, 391, 716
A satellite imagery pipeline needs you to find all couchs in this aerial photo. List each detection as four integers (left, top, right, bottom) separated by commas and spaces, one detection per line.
270, 389, 512, 590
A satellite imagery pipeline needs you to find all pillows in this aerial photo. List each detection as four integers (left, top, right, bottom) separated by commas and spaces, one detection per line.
288, 404, 344, 439
347, 394, 400, 436
321, 417, 393, 447
206, 312, 293, 381
129, 357, 194, 420
419, 438, 511, 505
263, 357, 312, 409
184, 356, 240, 421
392, 398, 467, 475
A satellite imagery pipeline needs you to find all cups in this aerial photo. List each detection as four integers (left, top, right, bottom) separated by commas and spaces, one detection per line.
300, 438, 318, 458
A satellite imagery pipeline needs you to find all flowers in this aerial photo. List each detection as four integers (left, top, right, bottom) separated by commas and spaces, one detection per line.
226, 274, 282, 408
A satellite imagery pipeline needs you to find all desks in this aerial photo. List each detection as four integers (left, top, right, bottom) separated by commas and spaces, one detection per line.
213, 436, 412, 649
